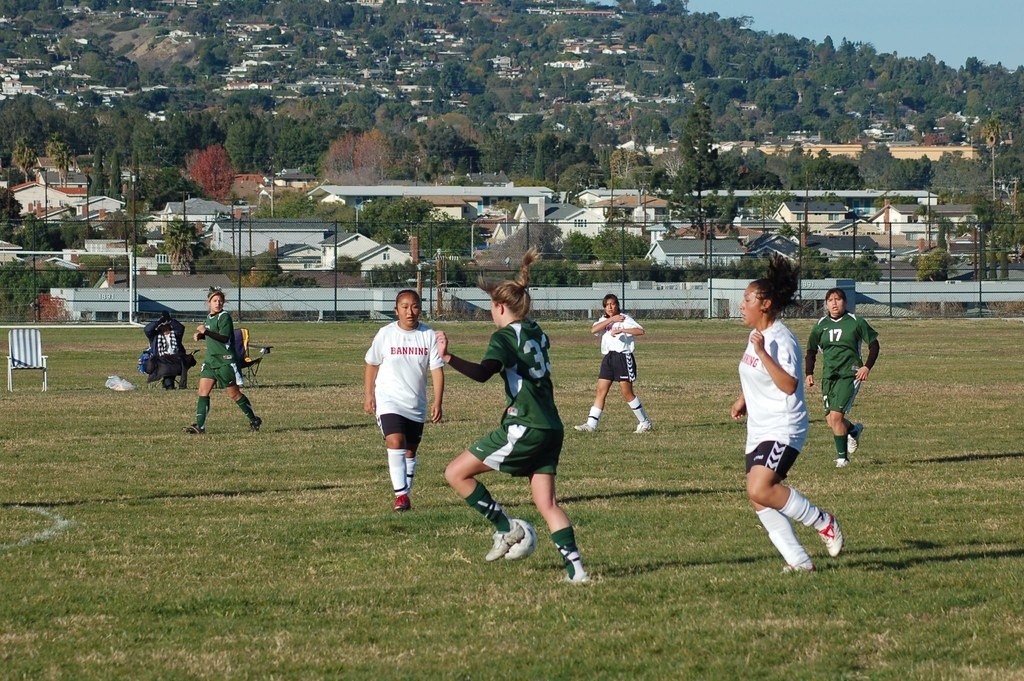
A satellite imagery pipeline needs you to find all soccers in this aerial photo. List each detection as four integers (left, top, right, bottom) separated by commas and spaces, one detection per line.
505, 519, 537, 560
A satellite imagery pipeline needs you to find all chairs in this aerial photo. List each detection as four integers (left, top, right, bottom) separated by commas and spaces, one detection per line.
5, 328, 49, 392
233, 327, 275, 390
142, 349, 200, 389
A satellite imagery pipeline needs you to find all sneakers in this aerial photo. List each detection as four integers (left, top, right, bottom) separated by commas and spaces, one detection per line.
633, 420, 652, 434
394, 494, 411, 512
557, 574, 590, 584
486, 521, 525, 561
574, 422, 598, 434
778, 556, 814, 575
814, 512, 843, 557
847, 423, 863, 453
832, 457, 850, 467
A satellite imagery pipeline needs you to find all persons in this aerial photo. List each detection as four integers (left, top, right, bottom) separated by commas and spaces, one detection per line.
804, 288, 880, 468
181, 286, 261, 432
143, 310, 188, 390
435, 250, 588, 583
730, 274, 843, 573
574, 293, 651, 433
364, 289, 445, 511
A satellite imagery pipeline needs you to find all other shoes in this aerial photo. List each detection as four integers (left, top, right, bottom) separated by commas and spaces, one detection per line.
247, 417, 262, 432
189, 423, 206, 434
